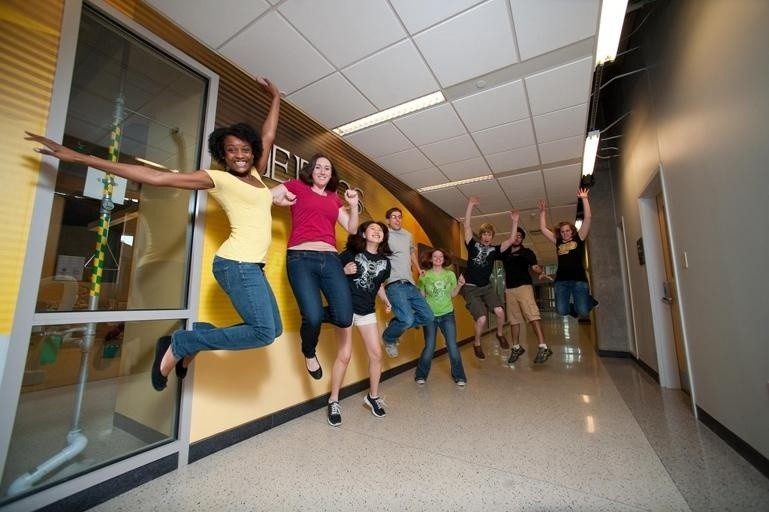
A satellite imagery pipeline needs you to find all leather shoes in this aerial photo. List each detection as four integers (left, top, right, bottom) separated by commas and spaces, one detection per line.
570, 303, 578, 318
151, 336, 173, 391
175, 358, 187, 378
592, 298, 599, 307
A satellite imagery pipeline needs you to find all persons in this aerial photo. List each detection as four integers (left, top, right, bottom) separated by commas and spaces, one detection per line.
264, 151, 361, 382
535, 186, 600, 321
494, 226, 555, 365
23, 74, 284, 392
325, 219, 394, 427
381, 207, 436, 359
96, 127, 191, 376
412, 246, 469, 390
461, 194, 520, 359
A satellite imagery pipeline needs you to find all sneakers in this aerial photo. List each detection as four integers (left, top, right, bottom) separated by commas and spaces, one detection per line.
305, 334, 552, 426
